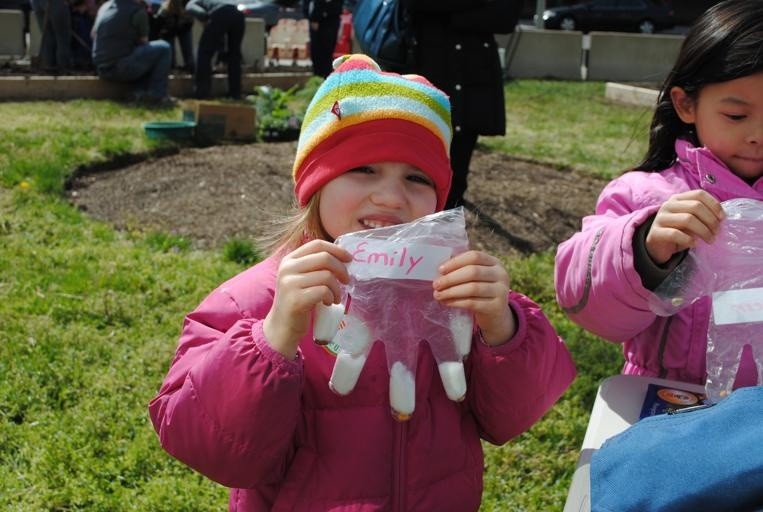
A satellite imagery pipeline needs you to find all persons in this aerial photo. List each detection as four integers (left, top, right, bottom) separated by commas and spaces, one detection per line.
147, 52, 577, 512
553, 1, 763, 390
347, 2, 538, 208
32, 1, 246, 101
303, 1, 342, 80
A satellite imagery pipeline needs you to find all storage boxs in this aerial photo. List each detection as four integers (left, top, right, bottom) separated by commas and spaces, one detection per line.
181, 98, 255, 142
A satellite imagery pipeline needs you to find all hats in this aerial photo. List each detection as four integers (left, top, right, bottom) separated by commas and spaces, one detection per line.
292, 54, 453, 213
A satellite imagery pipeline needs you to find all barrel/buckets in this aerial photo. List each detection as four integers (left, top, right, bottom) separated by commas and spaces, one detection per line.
144, 122, 198, 148
